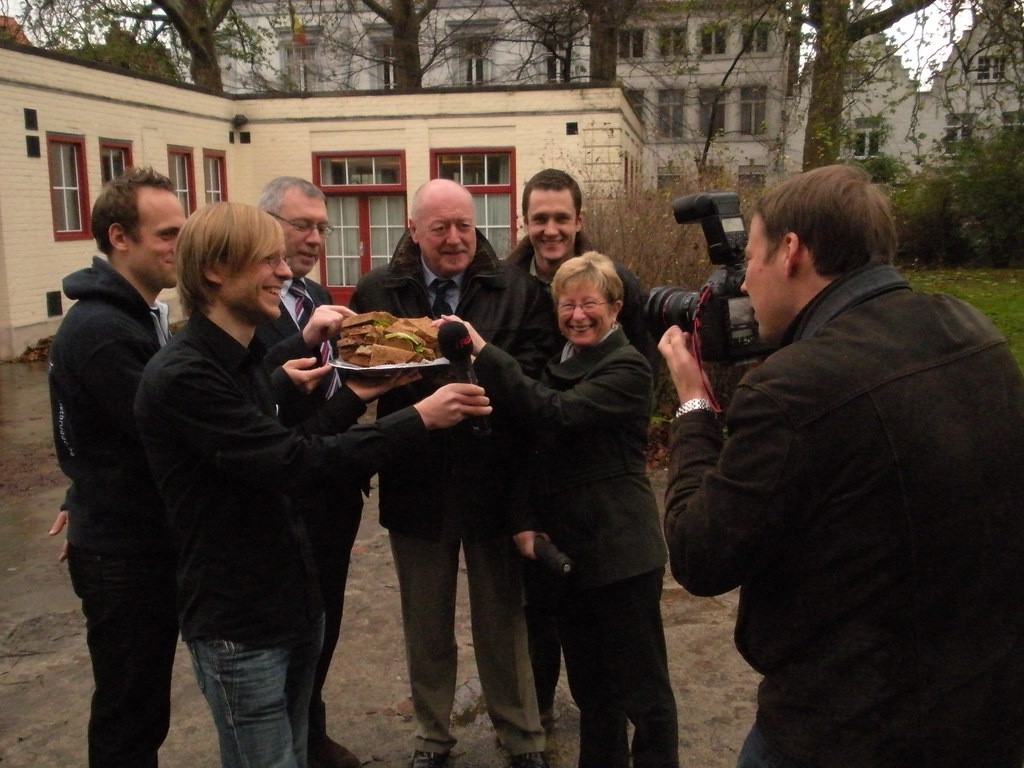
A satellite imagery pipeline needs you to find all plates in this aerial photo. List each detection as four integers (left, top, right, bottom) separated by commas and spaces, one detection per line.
327, 357, 450, 370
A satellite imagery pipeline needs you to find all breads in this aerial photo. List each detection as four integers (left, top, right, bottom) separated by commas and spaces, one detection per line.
337, 311, 442, 368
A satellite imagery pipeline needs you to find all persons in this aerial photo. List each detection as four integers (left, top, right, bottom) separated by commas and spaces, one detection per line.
48, 167, 492, 768
432, 252, 680, 768
501, 168, 650, 768
348, 177, 552, 768
656, 166, 1024, 768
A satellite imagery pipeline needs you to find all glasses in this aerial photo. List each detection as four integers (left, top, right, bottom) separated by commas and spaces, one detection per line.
557, 299, 610, 315
267, 211, 334, 238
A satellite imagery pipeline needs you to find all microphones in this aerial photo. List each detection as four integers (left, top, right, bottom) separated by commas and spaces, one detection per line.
437, 323, 492, 438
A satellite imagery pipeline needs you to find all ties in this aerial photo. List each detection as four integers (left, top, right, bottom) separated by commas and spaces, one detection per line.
288, 280, 342, 402
428, 280, 457, 321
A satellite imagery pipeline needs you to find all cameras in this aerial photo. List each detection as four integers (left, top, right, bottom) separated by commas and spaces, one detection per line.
642, 190, 781, 365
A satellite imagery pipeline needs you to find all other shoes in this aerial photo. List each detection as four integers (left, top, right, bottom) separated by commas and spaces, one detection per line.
540, 715, 560, 766
307, 733, 362, 768
412, 749, 450, 768
510, 752, 549, 768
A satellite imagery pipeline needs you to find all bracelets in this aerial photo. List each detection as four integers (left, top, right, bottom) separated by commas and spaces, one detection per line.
675, 398, 717, 418
